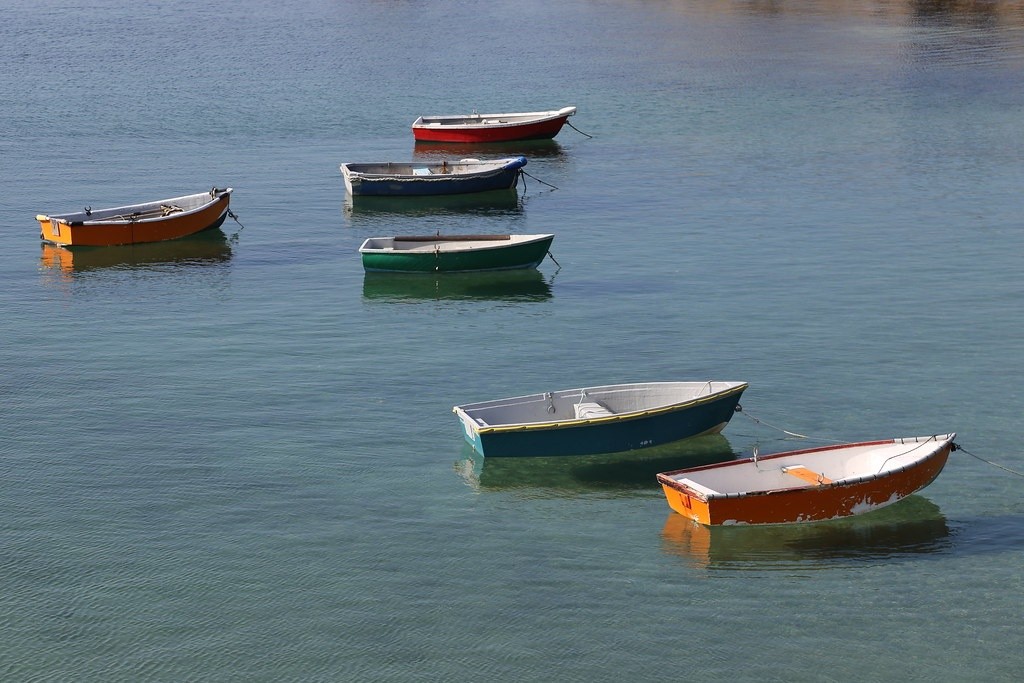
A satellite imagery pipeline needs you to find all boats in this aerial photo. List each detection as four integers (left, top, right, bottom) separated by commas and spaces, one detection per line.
361, 267, 555, 304
655, 432, 957, 528
413, 142, 567, 155
359, 230, 553, 275
660, 493, 956, 566
41, 230, 231, 269
460, 431, 736, 488
414, 106, 574, 141
451, 381, 749, 458
342, 189, 524, 219
341, 157, 526, 198
35, 186, 234, 248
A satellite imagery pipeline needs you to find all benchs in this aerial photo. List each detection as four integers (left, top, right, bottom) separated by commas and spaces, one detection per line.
782, 465, 834, 486
413, 168, 433, 175
573, 402, 613, 418
